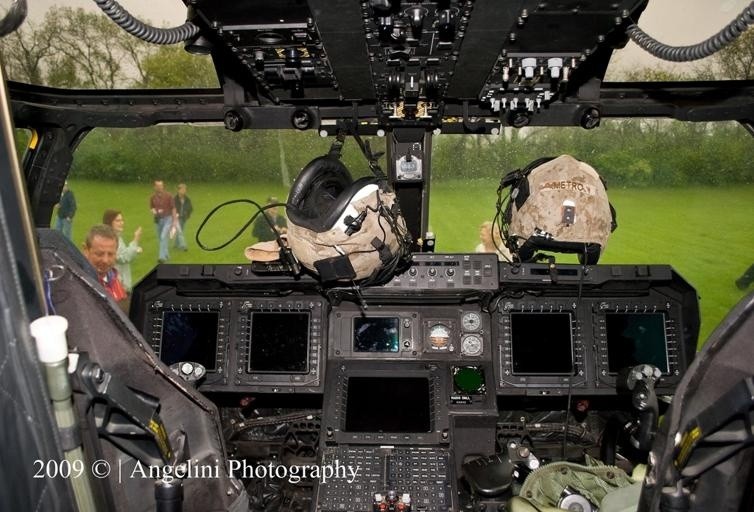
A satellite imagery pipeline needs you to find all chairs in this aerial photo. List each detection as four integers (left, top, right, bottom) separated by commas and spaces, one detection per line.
35, 227, 251, 512
599, 289, 754, 512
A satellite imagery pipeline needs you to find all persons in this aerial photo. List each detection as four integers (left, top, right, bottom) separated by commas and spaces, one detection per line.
82, 225, 128, 303
103, 209, 143, 292
475, 221, 513, 263
54, 181, 77, 240
252, 196, 287, 241
173, 183, 193, 250
150, 179, 177, 263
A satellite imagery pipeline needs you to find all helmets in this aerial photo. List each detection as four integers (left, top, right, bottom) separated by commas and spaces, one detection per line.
507, 155, 611, 266
284, 156, 407, 284
